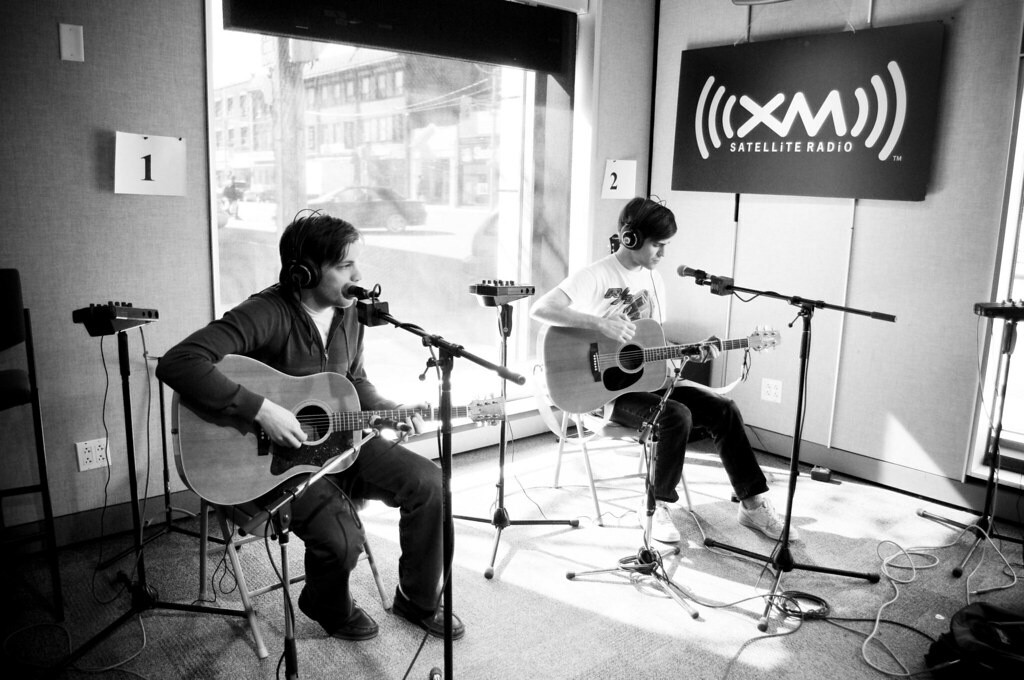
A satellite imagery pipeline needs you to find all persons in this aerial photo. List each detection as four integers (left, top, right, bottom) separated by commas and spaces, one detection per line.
155, 208, 465, 641
528, 197, 800, 542
227, 175, 241, 220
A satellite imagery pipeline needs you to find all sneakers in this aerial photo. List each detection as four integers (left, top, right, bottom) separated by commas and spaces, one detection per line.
738, 497, 798, 542
637, 498, 679, 542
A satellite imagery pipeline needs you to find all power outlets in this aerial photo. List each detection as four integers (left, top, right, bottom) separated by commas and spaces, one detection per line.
77, 437, 112, 471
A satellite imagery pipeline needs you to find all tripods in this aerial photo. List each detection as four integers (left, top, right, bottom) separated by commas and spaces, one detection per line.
451, 304, 580, 579
43, 332, 248, 680
696, 270, 895, 631
916, 320, 1023, 576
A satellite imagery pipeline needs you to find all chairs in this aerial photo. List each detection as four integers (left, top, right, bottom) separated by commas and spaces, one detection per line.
171, 388, 391, 658
540, 324, 692, 527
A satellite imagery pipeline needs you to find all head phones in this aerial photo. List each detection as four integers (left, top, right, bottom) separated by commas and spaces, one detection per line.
287, 209, 321, 290
620, 194, 666, 249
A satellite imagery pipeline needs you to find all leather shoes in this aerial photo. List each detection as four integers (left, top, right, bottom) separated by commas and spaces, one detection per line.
392, 584, 465, 640
298, 585, 379, 639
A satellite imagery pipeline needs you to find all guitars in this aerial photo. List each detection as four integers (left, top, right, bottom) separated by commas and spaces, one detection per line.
168, 351, 507, 508
543, 317, 784, 414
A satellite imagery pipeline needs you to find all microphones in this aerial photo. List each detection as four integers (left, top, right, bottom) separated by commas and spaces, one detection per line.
341, 283, 379, 300
677, 264, 717, 280
367, 415, 412, 431
